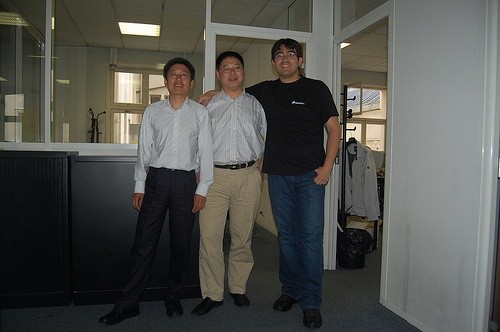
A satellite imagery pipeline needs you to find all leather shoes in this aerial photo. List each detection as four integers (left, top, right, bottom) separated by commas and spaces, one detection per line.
164, 296, 183, 317
229, 292, 250, 307
303, 309, 323, 328
273, 294, 297, 312
99, 305, 140, 325
191, 297, 224, 316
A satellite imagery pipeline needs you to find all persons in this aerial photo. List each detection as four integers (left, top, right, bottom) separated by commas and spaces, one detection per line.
195, 36, 341, 329
189, 50, 268, 317
97, 57, 216, 325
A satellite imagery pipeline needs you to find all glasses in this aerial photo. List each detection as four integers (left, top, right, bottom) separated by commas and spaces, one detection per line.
274, 52, 298, 60
219, 65, 243, 72
167, 73, 191, 79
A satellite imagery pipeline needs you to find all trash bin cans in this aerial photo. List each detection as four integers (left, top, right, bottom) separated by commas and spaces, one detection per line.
338, 228, 373, 272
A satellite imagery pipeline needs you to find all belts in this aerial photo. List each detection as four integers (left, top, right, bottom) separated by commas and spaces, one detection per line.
214, 160, 256, 170
